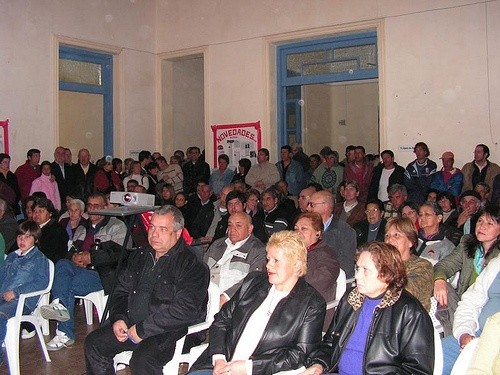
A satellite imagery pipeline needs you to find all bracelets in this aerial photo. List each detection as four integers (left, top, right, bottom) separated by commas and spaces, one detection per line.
434, 277, 446, 283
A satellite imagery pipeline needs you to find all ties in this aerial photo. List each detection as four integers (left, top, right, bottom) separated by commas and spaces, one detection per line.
249, 210, 253, 217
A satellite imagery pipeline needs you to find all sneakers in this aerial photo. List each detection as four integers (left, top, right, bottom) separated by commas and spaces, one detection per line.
39, 302, 70, 322
45, 329, 73, 351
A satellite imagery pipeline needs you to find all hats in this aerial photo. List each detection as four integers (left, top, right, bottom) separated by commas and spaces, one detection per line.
460, 190, 482, 200
439, 152, 454, 160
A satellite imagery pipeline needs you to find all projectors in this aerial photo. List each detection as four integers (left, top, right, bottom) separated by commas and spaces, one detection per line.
110, 191, 155, 208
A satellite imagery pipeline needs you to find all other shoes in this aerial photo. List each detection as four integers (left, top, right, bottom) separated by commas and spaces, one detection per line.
21, 329, 36, 339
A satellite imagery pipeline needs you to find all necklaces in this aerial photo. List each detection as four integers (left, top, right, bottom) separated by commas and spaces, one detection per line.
369, 227, 379, 232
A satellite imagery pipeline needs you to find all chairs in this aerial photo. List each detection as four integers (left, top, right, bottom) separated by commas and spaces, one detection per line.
0, 252, 460, 375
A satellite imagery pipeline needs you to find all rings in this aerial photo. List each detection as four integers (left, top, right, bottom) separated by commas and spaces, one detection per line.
227, 369, 232, 375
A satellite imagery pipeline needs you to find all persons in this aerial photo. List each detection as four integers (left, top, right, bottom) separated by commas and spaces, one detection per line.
0, 142, 500, 375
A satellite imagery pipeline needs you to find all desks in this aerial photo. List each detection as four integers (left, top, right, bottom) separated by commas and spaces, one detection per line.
88, 206, 162, 327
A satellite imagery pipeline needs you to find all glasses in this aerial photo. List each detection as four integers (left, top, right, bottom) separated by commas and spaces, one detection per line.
365, 208, 382, 213
298, 195, 310, 199
307, 201, 326, 207
88, 203, 105, 208
